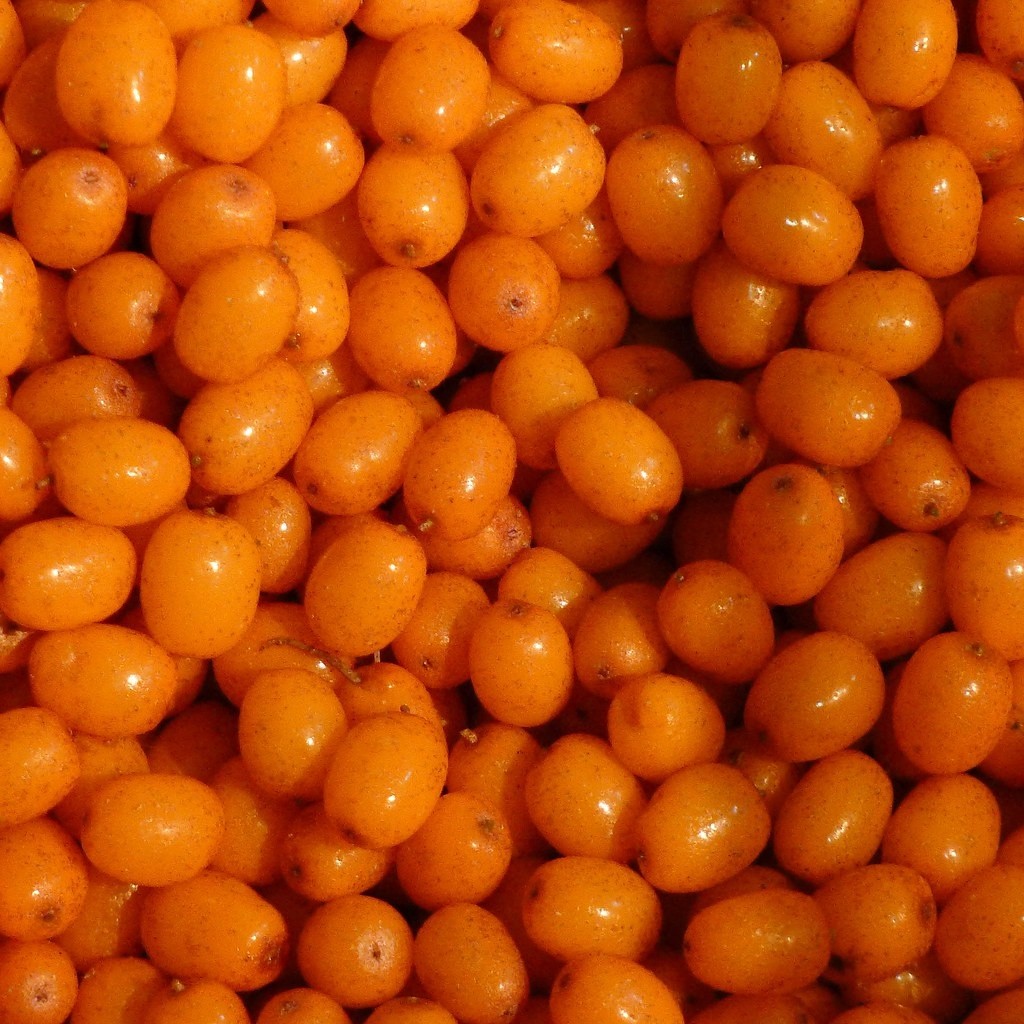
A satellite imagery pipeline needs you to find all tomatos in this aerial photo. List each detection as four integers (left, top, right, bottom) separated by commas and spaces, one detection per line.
0, 0, 1024, 1024
